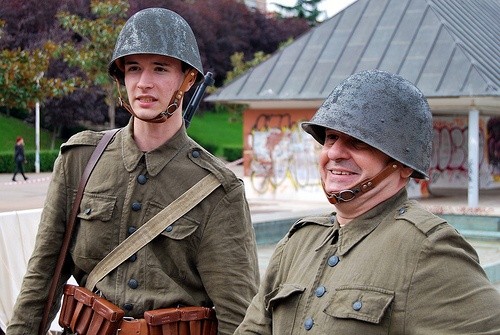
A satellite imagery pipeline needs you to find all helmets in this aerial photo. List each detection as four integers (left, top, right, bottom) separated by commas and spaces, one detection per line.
107, 8, 205, 87
301, 70, 433, 180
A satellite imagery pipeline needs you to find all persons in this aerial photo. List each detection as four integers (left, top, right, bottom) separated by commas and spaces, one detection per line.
232, 70, 500, 335
11, 136, 29, 182
6, 7, 261, 335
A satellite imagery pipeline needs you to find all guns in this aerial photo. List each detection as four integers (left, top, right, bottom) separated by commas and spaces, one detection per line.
183, 71, 216, 129
